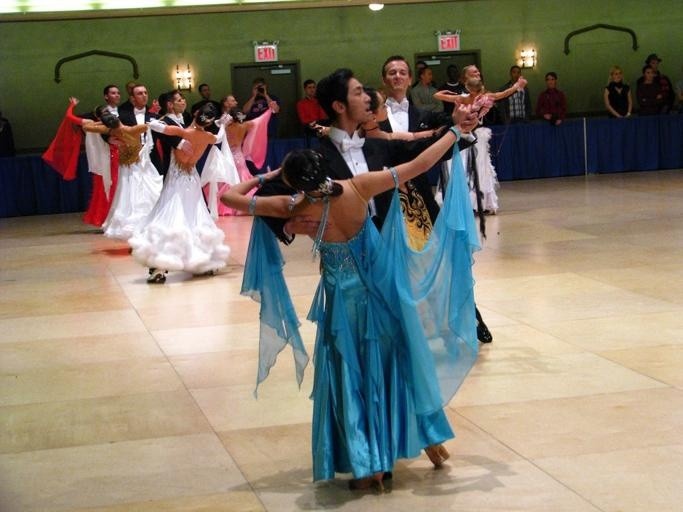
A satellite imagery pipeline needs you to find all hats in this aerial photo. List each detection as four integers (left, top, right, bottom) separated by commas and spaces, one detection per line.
644, 53, 662, 63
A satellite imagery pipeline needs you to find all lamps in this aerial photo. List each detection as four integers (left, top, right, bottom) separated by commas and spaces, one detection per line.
520, 49, 535, 68
175, 63, 192, 92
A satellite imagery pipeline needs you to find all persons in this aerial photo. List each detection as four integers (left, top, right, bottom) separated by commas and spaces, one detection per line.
220, 104, 471, 490
297, 79, 328, 138
43, 96, 120, 227
153, 90, 232, 176
129, 102, 230, 283
637, 54, 675, 113
443, 65, 481, 129
537, 72, 567, 124
255, 64, 479, 246
82, 112, 163, 242
119, 81, 148, 125
122, 86, 162, 175
433, 77, 527, 216
213, 95, 277, 133
498, 65, 532, 119
191, 84, 223, 132
202, 102, 275, 216
308, 88, 480, 356
149, 99, 161, 114
150, 90, 212, 274
412, 67, 444, 121
100, 85, 162, 177
241, 77, 280, 172
639, 66, 663, 112
383, 55, 493, 344
604, 65, 633, 117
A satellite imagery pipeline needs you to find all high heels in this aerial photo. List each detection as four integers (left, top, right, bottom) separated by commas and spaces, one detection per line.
425, 440, 451, 467
146, 268, 167, 282
348, 470, 387, 493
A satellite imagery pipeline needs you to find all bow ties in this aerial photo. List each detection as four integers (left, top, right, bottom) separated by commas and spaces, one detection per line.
391, 99, 410, 114
342, 137, 366, 154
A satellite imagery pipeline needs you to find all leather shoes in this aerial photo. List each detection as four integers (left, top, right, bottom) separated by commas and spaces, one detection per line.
475, 308, 493, 344
